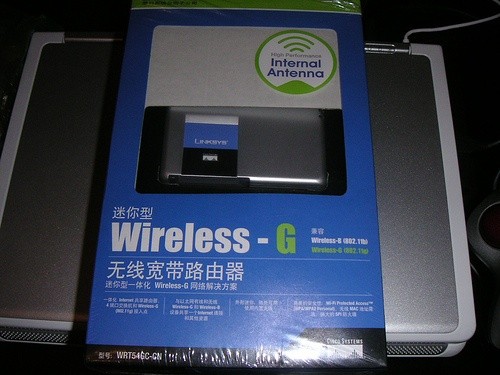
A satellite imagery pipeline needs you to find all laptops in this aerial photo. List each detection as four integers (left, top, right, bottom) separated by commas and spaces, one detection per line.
1, 33, 476, 357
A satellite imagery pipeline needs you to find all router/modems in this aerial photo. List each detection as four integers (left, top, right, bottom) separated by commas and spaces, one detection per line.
155, 107, 328, 194
